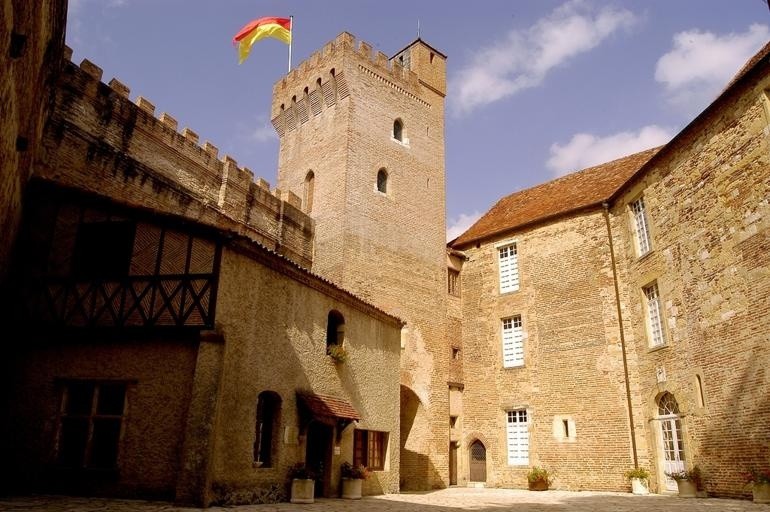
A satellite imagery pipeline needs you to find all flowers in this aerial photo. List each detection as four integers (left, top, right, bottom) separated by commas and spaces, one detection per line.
740, 466, 770, 491
327, 344, 348, 363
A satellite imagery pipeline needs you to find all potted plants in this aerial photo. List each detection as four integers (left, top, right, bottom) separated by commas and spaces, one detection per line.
340, 460, 367, 499
624, 468, 651, 495
665, 467, 702, 497
527, 466, 550, 490
288, 460, 317, 502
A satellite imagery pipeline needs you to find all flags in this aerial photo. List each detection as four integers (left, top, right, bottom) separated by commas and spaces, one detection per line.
232, 16, 291, 67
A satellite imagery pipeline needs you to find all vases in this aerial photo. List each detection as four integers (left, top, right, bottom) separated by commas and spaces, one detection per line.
751, 484, 770, 503
